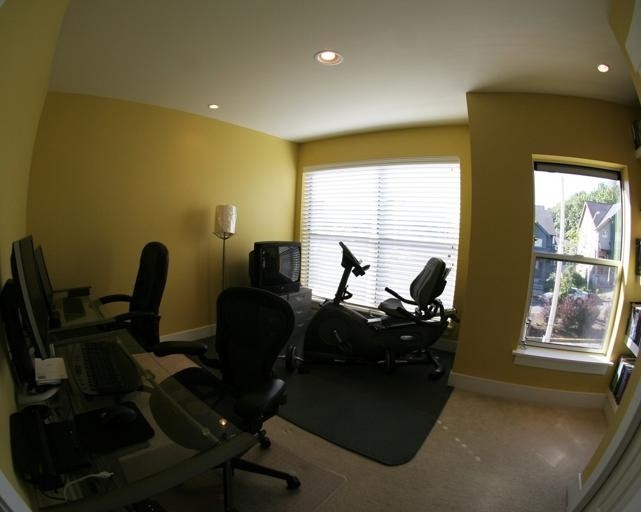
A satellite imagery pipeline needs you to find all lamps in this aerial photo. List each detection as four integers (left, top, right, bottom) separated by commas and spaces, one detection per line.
214, 204, 237, 291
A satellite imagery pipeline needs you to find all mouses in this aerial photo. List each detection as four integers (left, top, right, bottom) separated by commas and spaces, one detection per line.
99, 405, 137, 424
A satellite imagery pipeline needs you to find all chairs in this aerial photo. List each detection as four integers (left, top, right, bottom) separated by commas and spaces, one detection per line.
378, 257, 449, 325
148, 288, 302, 511
91, 240, 169, 375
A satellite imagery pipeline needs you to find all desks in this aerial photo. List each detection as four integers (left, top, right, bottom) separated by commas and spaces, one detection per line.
48, 285, 116, 339
18, 327, 258, 512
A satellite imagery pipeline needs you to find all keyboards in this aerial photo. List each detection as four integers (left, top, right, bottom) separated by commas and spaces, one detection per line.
71, 340, 141, 397
62, 296, 85, 317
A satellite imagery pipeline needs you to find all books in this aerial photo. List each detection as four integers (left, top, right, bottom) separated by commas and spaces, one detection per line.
608, 238, 641, 405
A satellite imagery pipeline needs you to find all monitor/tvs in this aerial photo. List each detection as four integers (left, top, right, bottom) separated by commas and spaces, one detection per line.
9, 234, 53, 360
248, 240, 301, 295
33, 244, 53, 300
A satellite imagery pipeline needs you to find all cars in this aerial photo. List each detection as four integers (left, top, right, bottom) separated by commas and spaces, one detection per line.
541, 286, 590, 306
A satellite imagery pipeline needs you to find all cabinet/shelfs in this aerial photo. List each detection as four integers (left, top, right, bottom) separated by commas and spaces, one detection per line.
278, 287, 312, 360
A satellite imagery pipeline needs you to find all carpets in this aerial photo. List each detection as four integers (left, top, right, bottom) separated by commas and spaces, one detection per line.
186, 332, 454, 466
